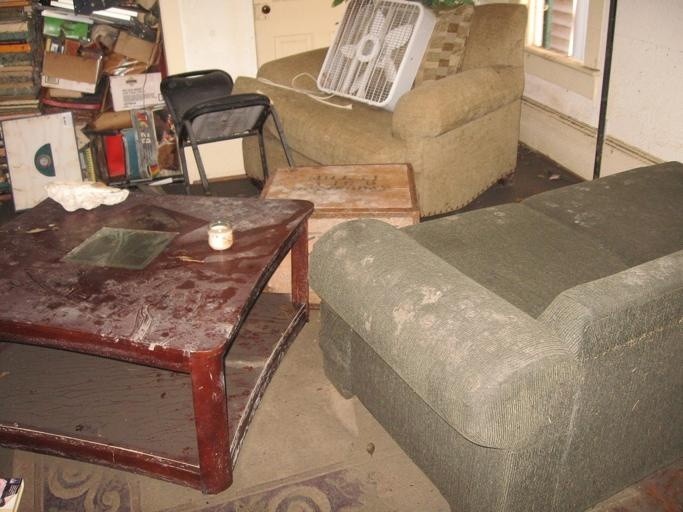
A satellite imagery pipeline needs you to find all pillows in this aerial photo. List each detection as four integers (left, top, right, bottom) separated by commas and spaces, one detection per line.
412, 4, 475, 85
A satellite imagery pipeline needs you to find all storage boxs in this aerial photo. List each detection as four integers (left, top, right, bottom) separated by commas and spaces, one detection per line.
106, 28, 159, 75
0, 43, 42, 100
257, 162, 420, 308
39, 10, 94, 40
0, 0, 42, 43
40, 46, 105, 95
108, 70, 162, 112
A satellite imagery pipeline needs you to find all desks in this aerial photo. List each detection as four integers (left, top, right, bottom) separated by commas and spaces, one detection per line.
1, 191, 315, 495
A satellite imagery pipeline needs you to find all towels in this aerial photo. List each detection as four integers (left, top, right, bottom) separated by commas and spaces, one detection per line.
43, 180, 129, 212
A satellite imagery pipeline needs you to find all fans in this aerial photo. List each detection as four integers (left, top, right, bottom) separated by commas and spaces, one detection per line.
316, 1, 438, 111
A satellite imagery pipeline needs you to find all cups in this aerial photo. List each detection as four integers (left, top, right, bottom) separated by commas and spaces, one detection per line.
205, 219, 232, 250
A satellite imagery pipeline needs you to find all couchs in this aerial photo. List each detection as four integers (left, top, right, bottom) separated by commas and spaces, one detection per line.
307, 160, 682, 511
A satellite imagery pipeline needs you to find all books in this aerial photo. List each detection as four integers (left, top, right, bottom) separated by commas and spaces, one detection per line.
0, 1, 45, 201
0, 476, 24, 511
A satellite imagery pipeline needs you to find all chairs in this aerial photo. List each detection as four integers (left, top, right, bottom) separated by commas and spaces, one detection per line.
232, 4, 528, 219
159, 68, 296, 196
